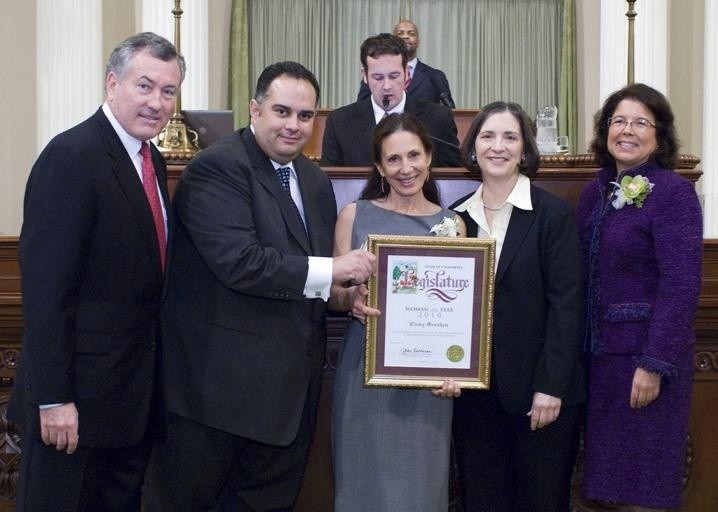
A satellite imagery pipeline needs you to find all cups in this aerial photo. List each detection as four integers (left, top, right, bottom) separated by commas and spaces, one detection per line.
557, 135, 570, 155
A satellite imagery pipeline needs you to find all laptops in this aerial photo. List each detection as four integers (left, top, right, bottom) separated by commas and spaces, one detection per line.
180, 110, 234, 149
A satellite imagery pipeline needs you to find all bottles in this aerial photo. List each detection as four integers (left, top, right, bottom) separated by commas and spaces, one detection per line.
535, 105, 558, 155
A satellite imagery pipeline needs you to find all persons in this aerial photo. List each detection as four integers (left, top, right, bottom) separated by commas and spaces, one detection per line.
573, 81, 701, 512
355, 19, 450, 105
329, 110, 466, 511
316, 31, 463, 168
141, 58, 375, 510
2, 31, 185, 511
447, 99, 584, 512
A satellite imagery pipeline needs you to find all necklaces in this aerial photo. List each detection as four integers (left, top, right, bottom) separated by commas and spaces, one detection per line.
481, 197, 500, 212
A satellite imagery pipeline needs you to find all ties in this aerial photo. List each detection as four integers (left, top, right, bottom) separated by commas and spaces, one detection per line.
403, 63, 412, 92
276, 167, 309, 243
135, 141, 167, 276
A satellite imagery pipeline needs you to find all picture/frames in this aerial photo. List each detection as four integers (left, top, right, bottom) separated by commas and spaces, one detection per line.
364, 233, 497, 390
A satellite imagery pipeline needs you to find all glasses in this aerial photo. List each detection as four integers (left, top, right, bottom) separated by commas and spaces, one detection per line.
607, 114, 658, 132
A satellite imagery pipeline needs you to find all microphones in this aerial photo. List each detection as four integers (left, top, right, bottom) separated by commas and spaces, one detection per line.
440, 92, 455, 108
383, 95, 481, 167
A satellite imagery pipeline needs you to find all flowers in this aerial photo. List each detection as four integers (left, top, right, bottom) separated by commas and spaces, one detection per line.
607, 175, 655, 210
